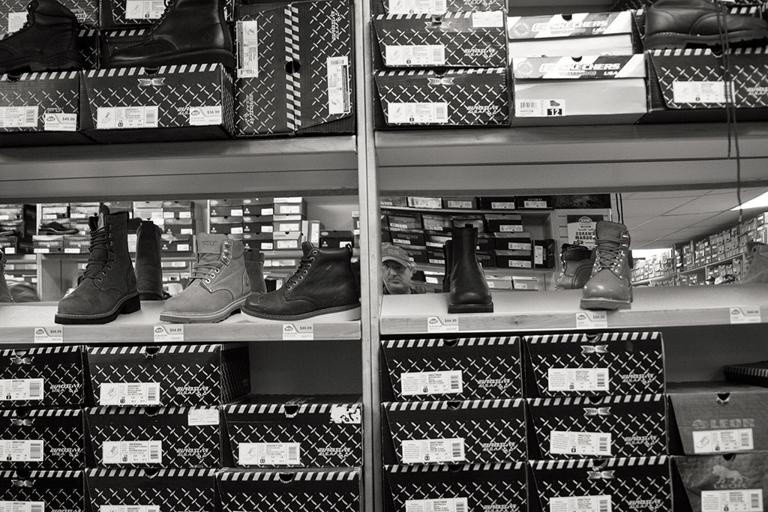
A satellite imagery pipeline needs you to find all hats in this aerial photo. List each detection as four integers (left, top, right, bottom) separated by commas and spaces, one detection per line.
381, 245, 408, 267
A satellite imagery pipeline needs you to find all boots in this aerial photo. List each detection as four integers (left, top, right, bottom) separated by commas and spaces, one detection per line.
134, 221, 163, 301
55, 212, 140, 325
558, 222, 631, 309
242, 243, 360, 325
242, 248, 268, 295
644, 0, 768, 49
110, 0, 234, 68
160, 232, 252, 324
443, 224, 493, 313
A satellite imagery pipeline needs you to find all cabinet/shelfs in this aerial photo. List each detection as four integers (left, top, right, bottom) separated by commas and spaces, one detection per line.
0, 0, 767, 512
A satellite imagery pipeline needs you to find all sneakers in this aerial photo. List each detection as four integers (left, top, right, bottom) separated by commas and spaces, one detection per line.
0, 0, 77, 71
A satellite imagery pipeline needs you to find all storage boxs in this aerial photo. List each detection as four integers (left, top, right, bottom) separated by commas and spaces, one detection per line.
0, 343, 363, 512
0, 195, 360, 287
369, 0, 767, 133
630, 213, 768, 291
379, 328, 768, 511
381, 193, 622, 292
1, 1, 358, 146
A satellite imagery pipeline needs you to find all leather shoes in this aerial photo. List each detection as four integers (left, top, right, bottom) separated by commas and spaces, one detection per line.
38, 222, 78, 235
